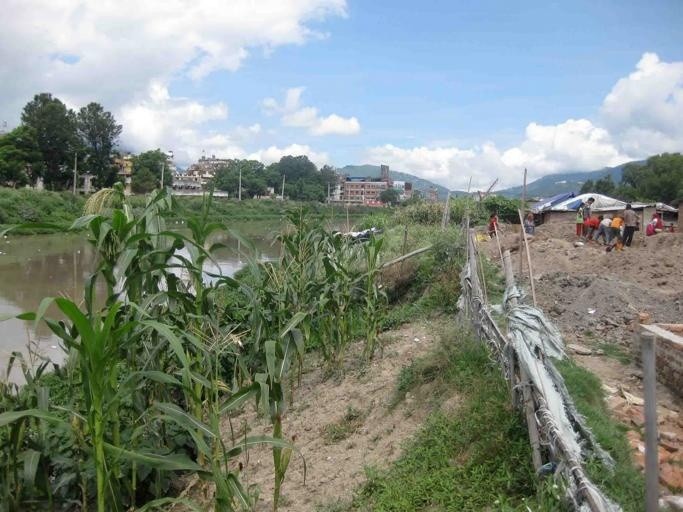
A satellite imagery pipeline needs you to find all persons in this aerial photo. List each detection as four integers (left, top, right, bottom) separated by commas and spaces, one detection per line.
487, 213, 501, 237
645, 213, 662, 237
524, 213, 535, 234
623, 203, 640, 246
668, 223, 674, 232
583, 198, 595, 239
575, 202, 585, 237
586, 215, 623, 245
